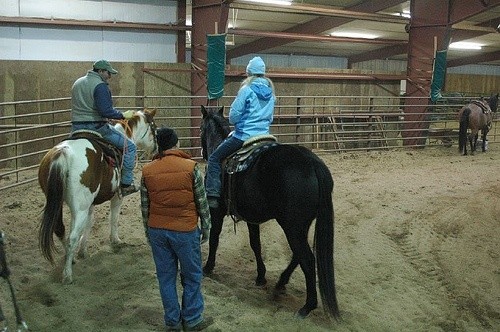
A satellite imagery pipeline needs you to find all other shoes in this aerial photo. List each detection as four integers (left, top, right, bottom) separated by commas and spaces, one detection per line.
167, 320, 182, 332
121, 185, 139, 197
206, 196, 219, 208
183, 316, 214, 332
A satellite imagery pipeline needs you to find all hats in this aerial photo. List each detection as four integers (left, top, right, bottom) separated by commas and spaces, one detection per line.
156, 127, 179, 150
245, 56, 265, 74
93, 60, 118, 74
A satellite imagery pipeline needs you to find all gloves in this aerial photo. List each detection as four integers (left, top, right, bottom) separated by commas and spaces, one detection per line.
199, 228, 209, 244
122, 110, 135, 118
146, 237, 151, 246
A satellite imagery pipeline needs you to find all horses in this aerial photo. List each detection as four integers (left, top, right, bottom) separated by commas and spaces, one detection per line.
38, 107, 162, 283
455, 93, 494, 155
199, 104, 342, 320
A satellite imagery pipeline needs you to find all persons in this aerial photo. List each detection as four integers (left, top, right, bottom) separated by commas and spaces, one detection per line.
205, 57, 275, 208
71, 59, 139, 196
140, 128, 213, 332
